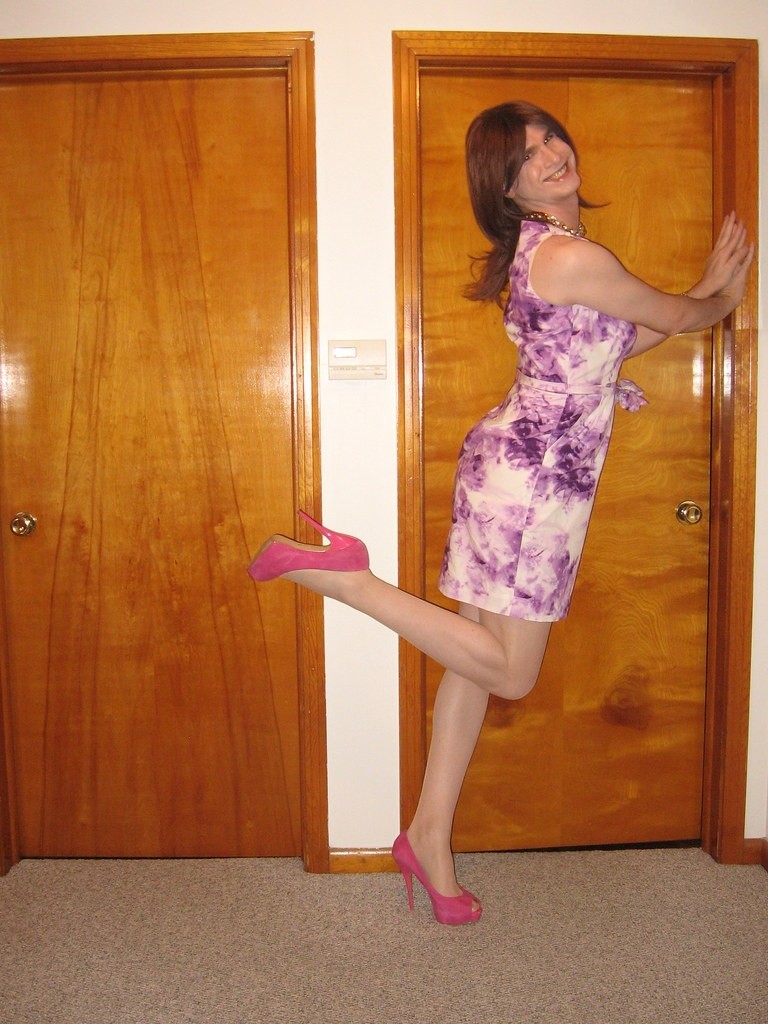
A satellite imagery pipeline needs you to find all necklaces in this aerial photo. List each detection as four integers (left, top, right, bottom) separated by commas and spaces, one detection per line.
526, 212, 586, 238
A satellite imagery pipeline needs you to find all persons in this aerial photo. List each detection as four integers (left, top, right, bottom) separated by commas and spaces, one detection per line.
248, 101, 755, 926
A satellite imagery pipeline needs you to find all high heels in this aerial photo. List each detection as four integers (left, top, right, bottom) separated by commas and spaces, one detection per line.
392, 827, 482, 928
247, 509, 369, 582
